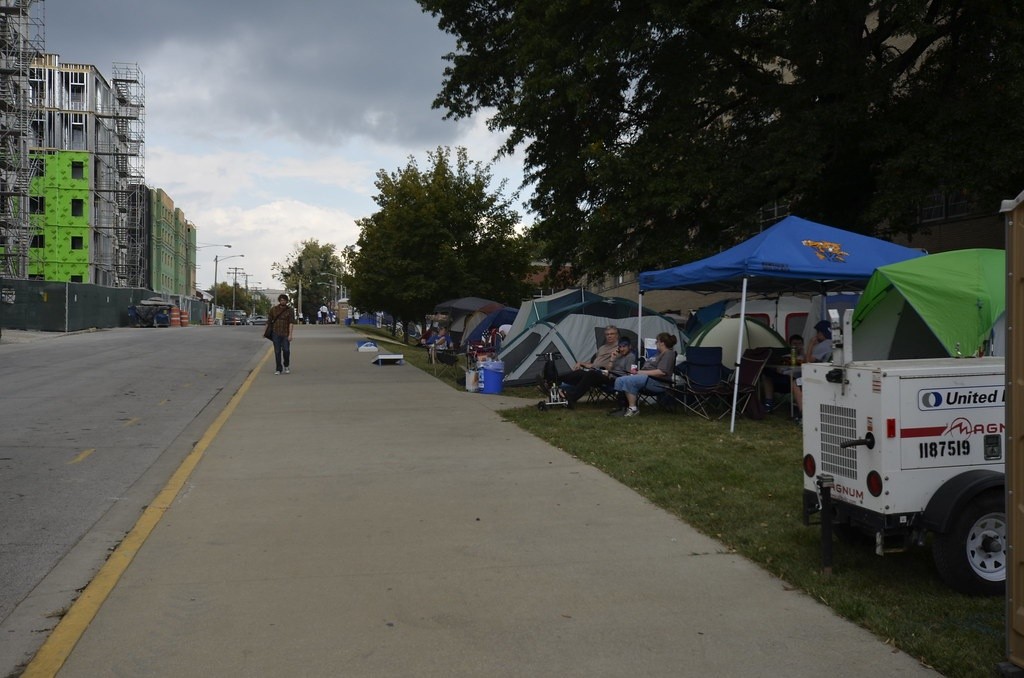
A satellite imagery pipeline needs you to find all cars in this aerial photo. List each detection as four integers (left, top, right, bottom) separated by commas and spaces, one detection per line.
246, 315, 268, 326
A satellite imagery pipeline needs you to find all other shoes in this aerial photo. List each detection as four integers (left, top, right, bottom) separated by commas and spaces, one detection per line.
283, 363, 290, 374
536, 384, 553, 397
550, 386, 561, 402
274, 370, 281, 375
558, 388, 571, 409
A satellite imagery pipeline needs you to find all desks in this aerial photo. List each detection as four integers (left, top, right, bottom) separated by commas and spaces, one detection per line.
758, 363, 801, 419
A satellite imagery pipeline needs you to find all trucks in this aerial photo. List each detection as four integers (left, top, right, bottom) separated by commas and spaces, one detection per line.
801, 248, 1007, 600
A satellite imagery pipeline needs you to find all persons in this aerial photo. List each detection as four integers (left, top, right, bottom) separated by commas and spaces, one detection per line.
537, 326, 621, 408
793, 320, 833, 421
320, 304, 328, 325
354, 311, 360, 324
499, 324, 512, 340
614, 333, 677, 417
607, 336, 636, 380
429, 326, 453, 353
298, 311, 303, 324
763, 334, 806, 412
269, 295, 295, 374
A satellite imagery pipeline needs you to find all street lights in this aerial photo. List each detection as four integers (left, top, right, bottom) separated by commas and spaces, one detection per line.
213, 255, 245, 325
320, 272, 337, 308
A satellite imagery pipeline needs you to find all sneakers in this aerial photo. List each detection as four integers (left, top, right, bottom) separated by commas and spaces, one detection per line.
623, 406, 640, 418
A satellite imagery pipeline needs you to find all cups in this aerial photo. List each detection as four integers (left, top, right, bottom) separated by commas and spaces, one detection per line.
631, 364, 637, 375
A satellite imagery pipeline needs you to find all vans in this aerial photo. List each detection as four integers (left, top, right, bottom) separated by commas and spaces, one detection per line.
224, 310, 246, 326
724, 296, 813, 346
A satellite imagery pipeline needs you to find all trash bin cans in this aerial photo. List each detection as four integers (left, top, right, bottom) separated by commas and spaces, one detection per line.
345, 318, 351, 326
476, 362, 505, 394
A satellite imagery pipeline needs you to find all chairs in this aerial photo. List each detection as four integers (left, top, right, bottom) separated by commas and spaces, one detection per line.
684, 346, 728, 416
424, 340, 459, 377
571, 345, 682, 414
466, 328, 498, 369
709, 348, 775, 423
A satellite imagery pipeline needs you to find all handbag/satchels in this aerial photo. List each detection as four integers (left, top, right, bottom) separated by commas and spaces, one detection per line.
264, 322, 273, 342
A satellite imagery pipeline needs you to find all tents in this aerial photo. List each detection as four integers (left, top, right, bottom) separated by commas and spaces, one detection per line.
852, 248, 1006, 361
433, 297, 519, 344
686, 315, 787, 369
495, 288, 693, 386
637, 216, 928, 432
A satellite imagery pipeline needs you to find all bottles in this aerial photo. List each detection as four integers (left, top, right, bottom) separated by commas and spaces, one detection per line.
791, 345, 797, 365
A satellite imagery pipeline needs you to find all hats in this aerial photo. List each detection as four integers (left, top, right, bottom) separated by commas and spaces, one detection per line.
815, 320, 832, 339
